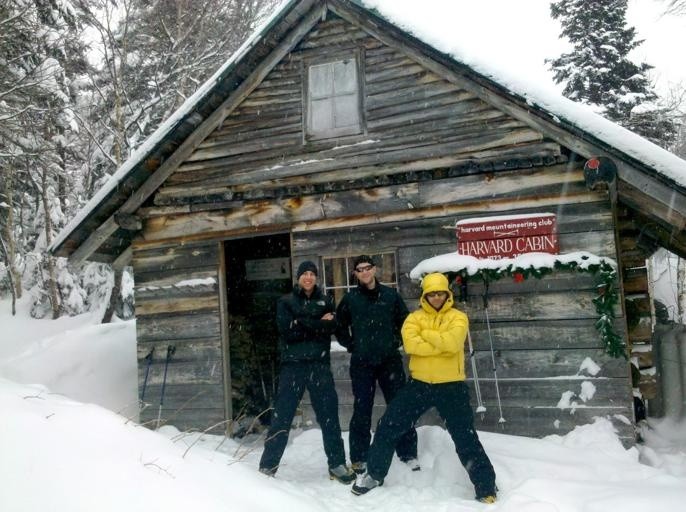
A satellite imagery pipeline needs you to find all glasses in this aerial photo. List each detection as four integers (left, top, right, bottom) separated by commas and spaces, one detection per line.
356, 265, 372, 271
428, 291, 445, 296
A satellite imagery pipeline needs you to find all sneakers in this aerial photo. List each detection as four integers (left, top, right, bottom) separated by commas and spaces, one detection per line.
401, 455, 420, 470
478, 495, 496, 504
329, 461, 384, 495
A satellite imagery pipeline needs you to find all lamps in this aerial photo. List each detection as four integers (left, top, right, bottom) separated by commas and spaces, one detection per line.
115, 213, 142, 230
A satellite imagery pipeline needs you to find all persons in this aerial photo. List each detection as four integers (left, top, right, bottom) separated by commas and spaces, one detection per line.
258, 260, 355, 483
351, 273, 498, 503
333, 255, 420, 475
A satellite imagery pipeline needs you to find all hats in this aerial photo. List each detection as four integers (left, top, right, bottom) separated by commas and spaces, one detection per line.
354, 255, 373, 270
297, 261, 317, 280
